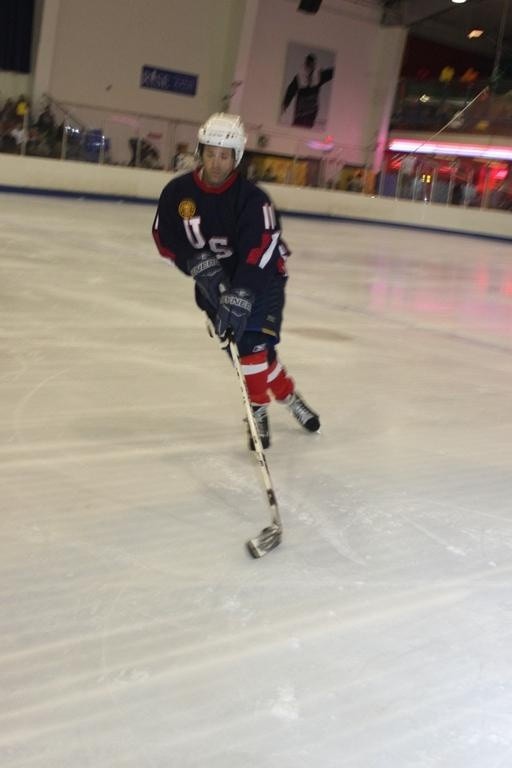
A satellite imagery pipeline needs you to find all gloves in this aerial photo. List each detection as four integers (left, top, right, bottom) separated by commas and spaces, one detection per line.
217, 286, 254, 342
191, 251, 227, 316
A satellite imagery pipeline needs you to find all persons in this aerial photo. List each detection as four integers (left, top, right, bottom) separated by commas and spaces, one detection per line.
170, 142, 195, 171
0, 95, 56, 157
251, 135, 277, 182
451, 182, 465, 203
151, 113, 320, 449
280, 52, 334, 128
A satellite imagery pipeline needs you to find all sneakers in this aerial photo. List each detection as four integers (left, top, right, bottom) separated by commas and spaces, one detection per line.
276, 392, 321, 432
248, 405, 270, 450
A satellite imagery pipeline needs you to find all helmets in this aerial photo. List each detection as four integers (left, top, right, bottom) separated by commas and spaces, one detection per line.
193, 113, 247, 169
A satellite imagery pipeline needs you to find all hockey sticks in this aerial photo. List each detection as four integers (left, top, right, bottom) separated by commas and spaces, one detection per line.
229, 341, 282, 558
